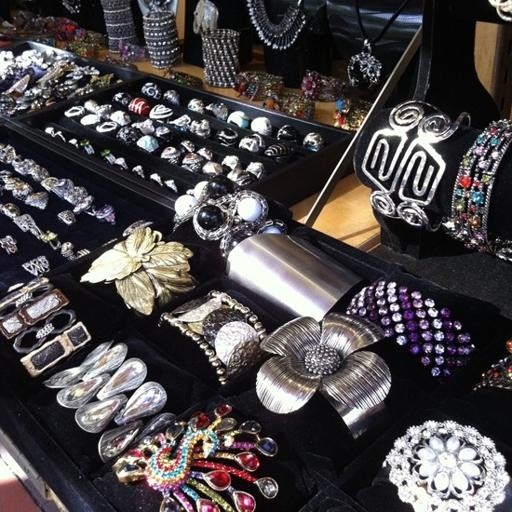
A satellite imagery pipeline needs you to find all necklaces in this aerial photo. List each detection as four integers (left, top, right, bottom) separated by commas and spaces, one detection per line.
247, 1, 306, 50
347, 0, 409, 91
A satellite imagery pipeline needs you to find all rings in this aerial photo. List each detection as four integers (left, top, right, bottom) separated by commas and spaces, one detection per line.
1, 82, 324, 294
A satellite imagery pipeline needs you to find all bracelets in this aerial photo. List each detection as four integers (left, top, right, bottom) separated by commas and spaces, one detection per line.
100, 0, 182, 69
362, 99, 512, 260
199, 29, 243, 89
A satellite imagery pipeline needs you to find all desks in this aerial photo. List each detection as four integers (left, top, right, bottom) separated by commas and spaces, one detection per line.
1, 31, 512, 511
